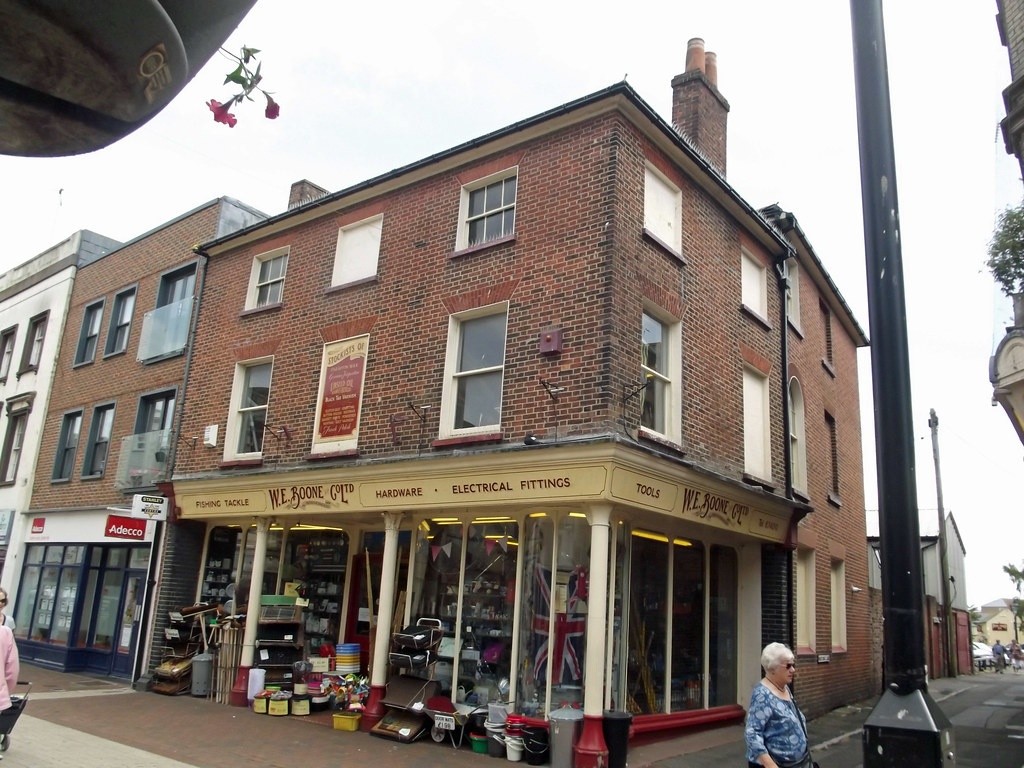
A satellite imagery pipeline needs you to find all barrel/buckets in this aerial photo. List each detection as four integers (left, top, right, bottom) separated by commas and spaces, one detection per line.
602, 709, 632, 768
468, 703, 549, 765
192, 652, 217, 695
547, 705, 584, 768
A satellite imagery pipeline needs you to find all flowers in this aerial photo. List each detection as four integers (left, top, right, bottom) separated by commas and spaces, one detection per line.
190, 27, 281, 128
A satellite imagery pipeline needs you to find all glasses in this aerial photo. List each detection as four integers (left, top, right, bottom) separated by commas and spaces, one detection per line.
0, 598, 7, 604
780, 662, 797, 669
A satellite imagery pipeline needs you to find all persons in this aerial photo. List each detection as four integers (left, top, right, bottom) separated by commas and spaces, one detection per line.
992, 640, 1006, 674
1008, 639, 1024, 672
744, 641, 810, 768
0, 588, 20, 759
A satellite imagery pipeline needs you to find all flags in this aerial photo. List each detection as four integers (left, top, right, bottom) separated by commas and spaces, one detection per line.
529, 563, 588, 688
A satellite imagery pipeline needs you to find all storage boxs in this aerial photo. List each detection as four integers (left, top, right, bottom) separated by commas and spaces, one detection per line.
332, 711, 362, 732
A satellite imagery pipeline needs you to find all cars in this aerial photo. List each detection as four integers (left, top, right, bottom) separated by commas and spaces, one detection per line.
973, 641, 1024, 665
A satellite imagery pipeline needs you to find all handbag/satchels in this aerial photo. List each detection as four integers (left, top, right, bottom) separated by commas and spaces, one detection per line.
775, 740, 813, 768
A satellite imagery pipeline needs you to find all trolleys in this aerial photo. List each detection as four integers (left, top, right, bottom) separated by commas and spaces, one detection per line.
0, 681, 33, 753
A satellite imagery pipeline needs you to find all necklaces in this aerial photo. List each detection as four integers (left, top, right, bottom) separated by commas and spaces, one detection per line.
765, 676, 783, 692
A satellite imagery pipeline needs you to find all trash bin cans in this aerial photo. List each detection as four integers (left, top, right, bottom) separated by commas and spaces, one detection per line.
190, 654, 212, 696
603, 710, 633, 768
546, 705, 583, 768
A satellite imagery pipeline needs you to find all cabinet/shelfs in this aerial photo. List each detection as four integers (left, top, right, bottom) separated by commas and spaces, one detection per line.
151, 528, 513, 744
